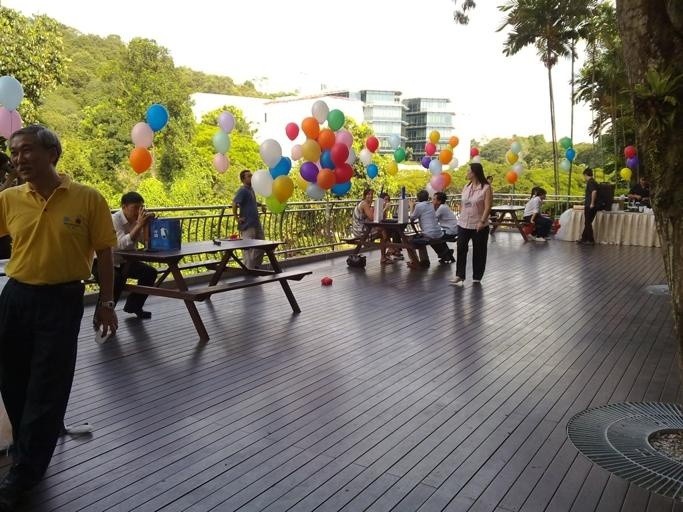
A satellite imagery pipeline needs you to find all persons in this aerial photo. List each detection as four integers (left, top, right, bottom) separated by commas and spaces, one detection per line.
532, 188, 539, 196
487, 176, 493, 184
430, 192, 458, 263
523, 189, 552, 243
575, 167, 599, 246
0, 136, 8, 152
402, 189, 445, 268
231, 170, 270, 274
626, 176, 650, 208
1, 124, 120, 503
352, 188, 404, 265
90, 190, 156, 328
378, 192, 404, 262
1, 152, 24, 191
449, 162, 493, 283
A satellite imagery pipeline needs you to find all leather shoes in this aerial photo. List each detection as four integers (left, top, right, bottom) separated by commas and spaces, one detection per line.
124, 304, 151, 319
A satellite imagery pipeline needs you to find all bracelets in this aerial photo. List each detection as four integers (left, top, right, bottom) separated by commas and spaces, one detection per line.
97, 301, 115, 310
480, 221, 486, 225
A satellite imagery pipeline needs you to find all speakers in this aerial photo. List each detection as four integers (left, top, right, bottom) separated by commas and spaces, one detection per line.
598, 182, 615, 210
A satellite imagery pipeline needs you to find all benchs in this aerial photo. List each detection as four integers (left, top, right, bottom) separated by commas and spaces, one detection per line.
405, 236, 457, 271
83, 258, 247, 295
520, 221, 534, 225
184, 270, 313, 340
340, 231, 382, 246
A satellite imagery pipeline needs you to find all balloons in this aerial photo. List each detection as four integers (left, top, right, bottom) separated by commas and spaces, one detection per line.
565, 148, 575, 163
213, 134, 230, 155
473, 154, 480, 164
219, 111, 234, 134
130, 122, 153, 149
0, 76, 25, 113
559, 136, 571, 149
250, 97, 358, 216
505, 141, 524, 186
212, 153, 228, 175
624, 145, 636, 157
0, 106, 22, 140
626, 155, 639, 169
620, 167, 633, 180
147, 104, 168, 132
129, 148, 151, 174
422, 130, 461, 197
471, 148, 479, 157
359, 135, 406, 180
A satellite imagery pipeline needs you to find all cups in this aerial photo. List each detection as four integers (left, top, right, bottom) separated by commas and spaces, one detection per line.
93, 324, 113, 345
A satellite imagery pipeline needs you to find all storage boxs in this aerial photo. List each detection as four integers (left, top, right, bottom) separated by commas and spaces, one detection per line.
149, 219, 181, 251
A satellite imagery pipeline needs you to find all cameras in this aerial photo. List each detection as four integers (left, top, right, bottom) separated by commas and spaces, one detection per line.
141, 212, 155, 221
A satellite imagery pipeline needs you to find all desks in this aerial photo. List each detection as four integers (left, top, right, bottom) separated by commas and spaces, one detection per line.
114, 237, 301, 339
554, 202, 662, 247
490, 205, 528, 242
352, 221, 422, 263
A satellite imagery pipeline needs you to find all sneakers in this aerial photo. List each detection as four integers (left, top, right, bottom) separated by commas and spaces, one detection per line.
473, 279, 481, 282
576, 238, 595, 246
529, 233, 551, 242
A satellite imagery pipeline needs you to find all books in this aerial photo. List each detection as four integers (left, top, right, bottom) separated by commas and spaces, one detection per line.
561, 157, 571, 173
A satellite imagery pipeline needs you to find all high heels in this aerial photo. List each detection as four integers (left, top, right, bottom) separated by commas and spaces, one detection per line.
448, 275, 464, 284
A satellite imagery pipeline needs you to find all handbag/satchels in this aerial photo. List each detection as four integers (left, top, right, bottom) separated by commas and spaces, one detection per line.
347, 254, 366, 268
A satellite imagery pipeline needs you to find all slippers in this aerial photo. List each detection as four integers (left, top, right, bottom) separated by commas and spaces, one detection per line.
381, 248, 403, 264
